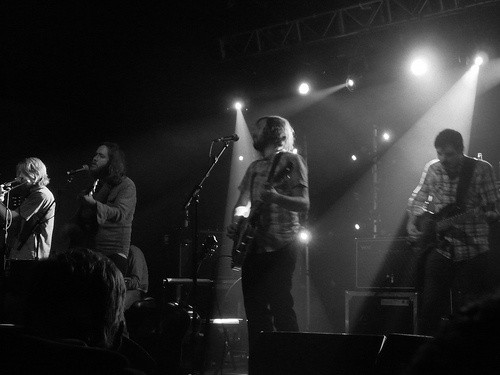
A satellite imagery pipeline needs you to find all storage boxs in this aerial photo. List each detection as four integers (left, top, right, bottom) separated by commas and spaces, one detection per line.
344, 291, 424, 337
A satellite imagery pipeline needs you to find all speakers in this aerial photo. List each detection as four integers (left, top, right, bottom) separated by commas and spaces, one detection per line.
257, 330, 446, 375
355, 238, 427, 290
346, 288, 416, 333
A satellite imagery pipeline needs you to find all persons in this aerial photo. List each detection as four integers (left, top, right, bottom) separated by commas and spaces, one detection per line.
226, 115, 309, 352
0, 157, 55, 322
78, 141, 137, 278
124, 245, 147, 309
20, 247, 127, 353
406, 128, 500, 336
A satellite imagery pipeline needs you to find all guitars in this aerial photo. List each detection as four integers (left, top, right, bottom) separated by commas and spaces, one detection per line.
231, 146, 285, 270
158, 235, 219, 375
408, 203, 495, 257
70, 179, 100, 242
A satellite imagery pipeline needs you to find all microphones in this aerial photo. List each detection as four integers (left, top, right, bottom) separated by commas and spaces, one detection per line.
67, 164, 88, 175
215, 135, 238, 141
